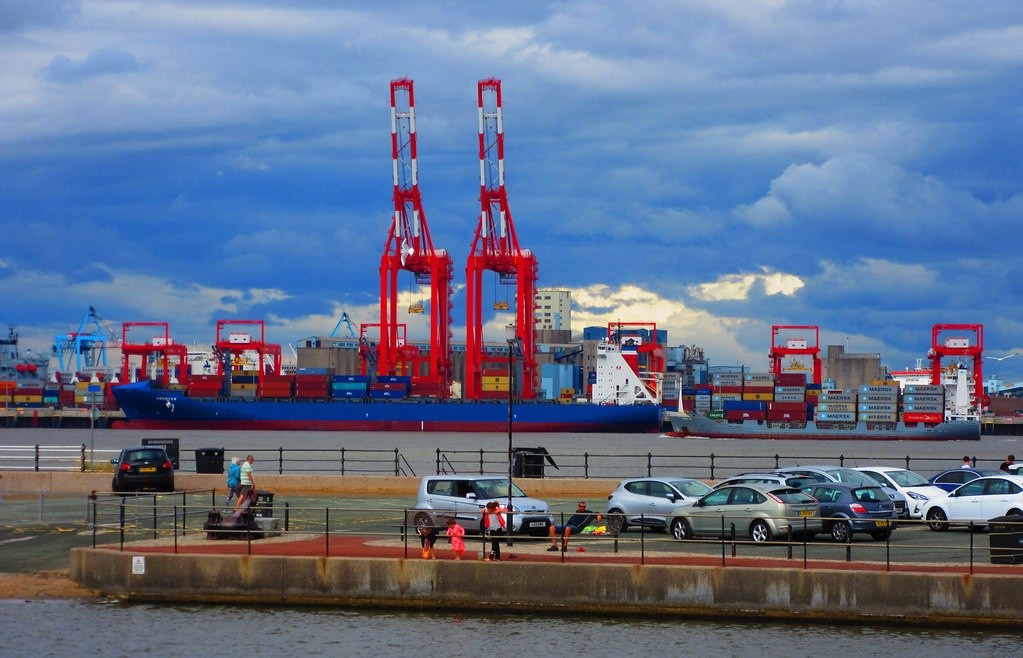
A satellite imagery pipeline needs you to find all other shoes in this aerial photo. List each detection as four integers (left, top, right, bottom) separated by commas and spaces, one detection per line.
562, 546, 567, 551
233, 508, 238, 513
547, 546, 558, 551
223, 509, 231, 513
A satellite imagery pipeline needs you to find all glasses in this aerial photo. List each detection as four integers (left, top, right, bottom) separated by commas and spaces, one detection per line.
250, 459, 253, 461
579, 506, 586, 508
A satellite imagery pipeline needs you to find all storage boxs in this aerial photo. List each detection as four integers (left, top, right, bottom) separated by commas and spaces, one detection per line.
482, 368, 596, 403
0, 380, 121, 410
185, 369, 448, 399
662, 374, 945, 423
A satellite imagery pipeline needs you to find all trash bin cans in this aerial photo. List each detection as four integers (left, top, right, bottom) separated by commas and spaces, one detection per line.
514, 446, 559, 479
255, 491, 273, 515
989, 516, 1023, 565
194, 447, 225, 473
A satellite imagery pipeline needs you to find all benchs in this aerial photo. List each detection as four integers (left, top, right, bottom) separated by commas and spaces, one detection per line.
570, 515, 624, 554
392, 508, 458, 545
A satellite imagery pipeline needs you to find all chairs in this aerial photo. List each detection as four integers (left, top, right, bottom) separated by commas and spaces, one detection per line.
655, 484, 666, 496
791, 481, 802, 488
861, 493, 870, 499
835, 493, 841, 500
736, 491, 751, 502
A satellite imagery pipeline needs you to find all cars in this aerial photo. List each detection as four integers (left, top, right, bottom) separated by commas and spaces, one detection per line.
927, 467, 1019, 495
920, 474, 1023, 533
710, 471, 820, 489
766, 465, 909, 521
667, 482, 824, 546
793, 482, 898, 544
847, 466, 950, 522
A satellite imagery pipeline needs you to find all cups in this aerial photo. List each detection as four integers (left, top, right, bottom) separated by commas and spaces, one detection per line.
478, 552, 483, 561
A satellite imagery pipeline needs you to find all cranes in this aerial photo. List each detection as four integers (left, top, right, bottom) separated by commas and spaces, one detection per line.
464, 75, 543, 400
331, 311, 362, 339
51, 304, 123, 383
378, 76, 455, 387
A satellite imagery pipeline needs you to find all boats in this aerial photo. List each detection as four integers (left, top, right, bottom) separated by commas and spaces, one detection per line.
662, 416, 984, 440
109, 379, 683, 433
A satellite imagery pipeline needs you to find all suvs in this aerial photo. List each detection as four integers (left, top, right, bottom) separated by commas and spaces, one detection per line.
418, 474, 554, 542
607, 475, 729, 536
110, 445, 175, 497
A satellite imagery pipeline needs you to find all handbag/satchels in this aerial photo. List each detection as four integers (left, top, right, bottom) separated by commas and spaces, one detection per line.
580, 524, 597, 535
595, 526, 606, 533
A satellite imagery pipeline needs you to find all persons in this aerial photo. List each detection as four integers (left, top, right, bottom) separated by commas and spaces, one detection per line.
446, 519, 465, 560
421, 528, 439, 560
233, 455, 256, 512
547, 502, 602, 552
482, 502, 508, 560
1000, 455, 1015, 473
223, 458, 241, 512
960, 456, 972, 468
